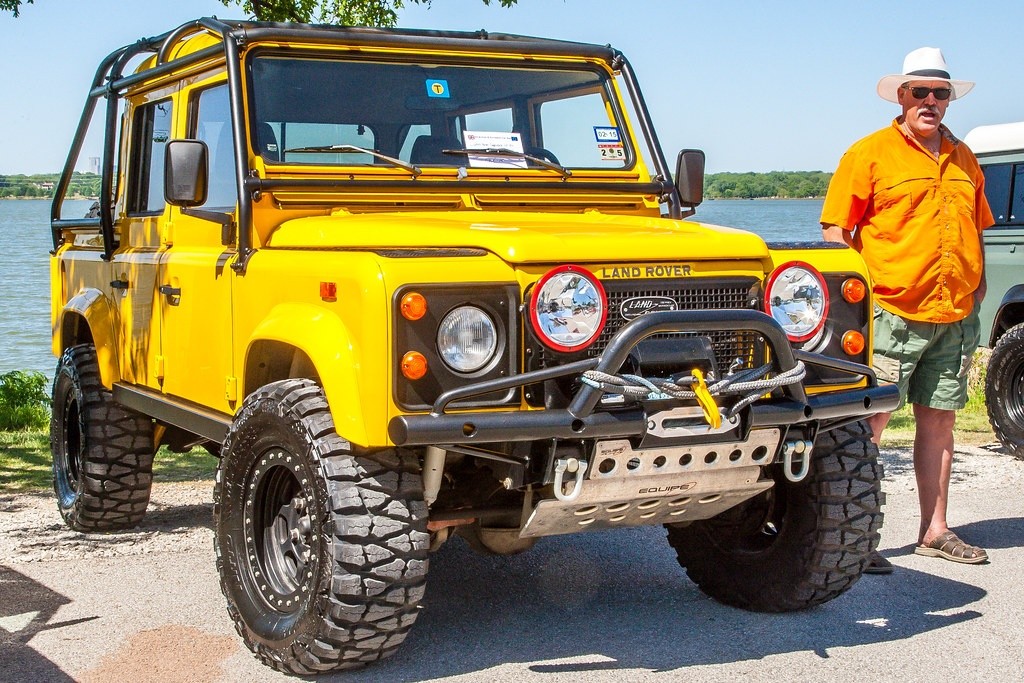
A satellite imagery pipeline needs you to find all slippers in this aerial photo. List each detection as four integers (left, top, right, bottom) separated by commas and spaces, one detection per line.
862, 549, 895, 574
914, 529, 989, 564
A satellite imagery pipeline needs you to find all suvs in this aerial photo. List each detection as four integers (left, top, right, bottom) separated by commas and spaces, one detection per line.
958, 122, 1023, 459
48, 12, 899, 681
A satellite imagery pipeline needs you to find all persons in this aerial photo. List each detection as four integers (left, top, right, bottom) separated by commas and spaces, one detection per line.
821, 47, 988, 574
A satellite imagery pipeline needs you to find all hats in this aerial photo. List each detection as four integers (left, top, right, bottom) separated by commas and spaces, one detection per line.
877, 46, 975, 104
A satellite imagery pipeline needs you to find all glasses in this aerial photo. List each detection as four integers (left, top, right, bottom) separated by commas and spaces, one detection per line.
901, 85, 952, 100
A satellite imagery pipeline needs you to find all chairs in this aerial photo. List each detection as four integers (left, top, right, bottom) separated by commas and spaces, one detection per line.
213, 118, 279, 197
409, 135, 468, 165
